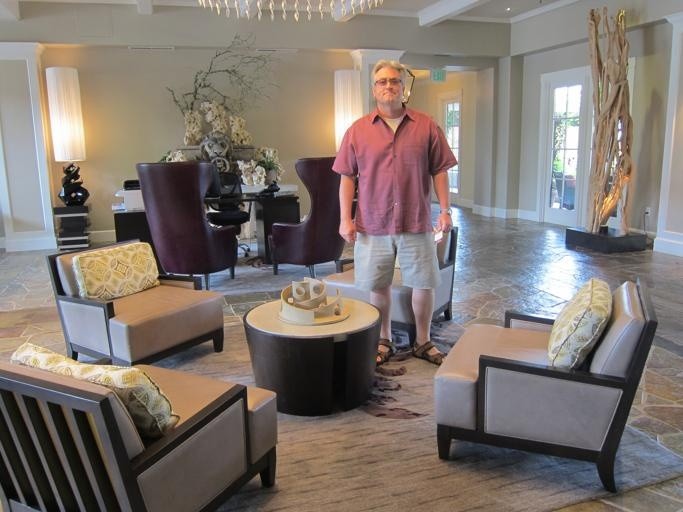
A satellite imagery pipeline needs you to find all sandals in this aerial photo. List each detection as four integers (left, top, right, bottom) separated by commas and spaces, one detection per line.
375, 338, 395, 364
412, 340, 447, 366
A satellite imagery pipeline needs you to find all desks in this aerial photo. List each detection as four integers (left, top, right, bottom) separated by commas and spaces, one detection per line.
52, 202, 92, 250
112, 194, 302, 266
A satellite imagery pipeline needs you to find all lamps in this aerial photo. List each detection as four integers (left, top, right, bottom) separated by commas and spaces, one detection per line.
42, 66, 89, 206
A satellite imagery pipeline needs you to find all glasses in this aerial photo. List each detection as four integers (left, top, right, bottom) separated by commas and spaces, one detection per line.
375, 77, 402, 84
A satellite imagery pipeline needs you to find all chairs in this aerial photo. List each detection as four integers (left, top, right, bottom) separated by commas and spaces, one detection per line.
268, 156, 356, 277
133, 161, 238, 293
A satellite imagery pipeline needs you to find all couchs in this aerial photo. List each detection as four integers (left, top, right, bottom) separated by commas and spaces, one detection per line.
1, 341, 278, 512
43, 238, 224, 367
434, 273, 659, 491
324, 224, 459, 325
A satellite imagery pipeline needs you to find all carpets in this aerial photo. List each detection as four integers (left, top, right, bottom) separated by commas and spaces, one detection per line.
1, 312, 682, 512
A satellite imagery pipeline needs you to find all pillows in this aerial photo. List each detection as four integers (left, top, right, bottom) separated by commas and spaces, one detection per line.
70, 241, 159, 302
548, 277, 611, 372
8, 342, 176, 437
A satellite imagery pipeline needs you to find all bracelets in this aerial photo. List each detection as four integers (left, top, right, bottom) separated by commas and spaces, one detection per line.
438, 208, 452, 216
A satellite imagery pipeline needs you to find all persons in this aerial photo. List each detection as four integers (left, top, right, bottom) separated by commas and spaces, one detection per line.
328, 58, 455, 370
